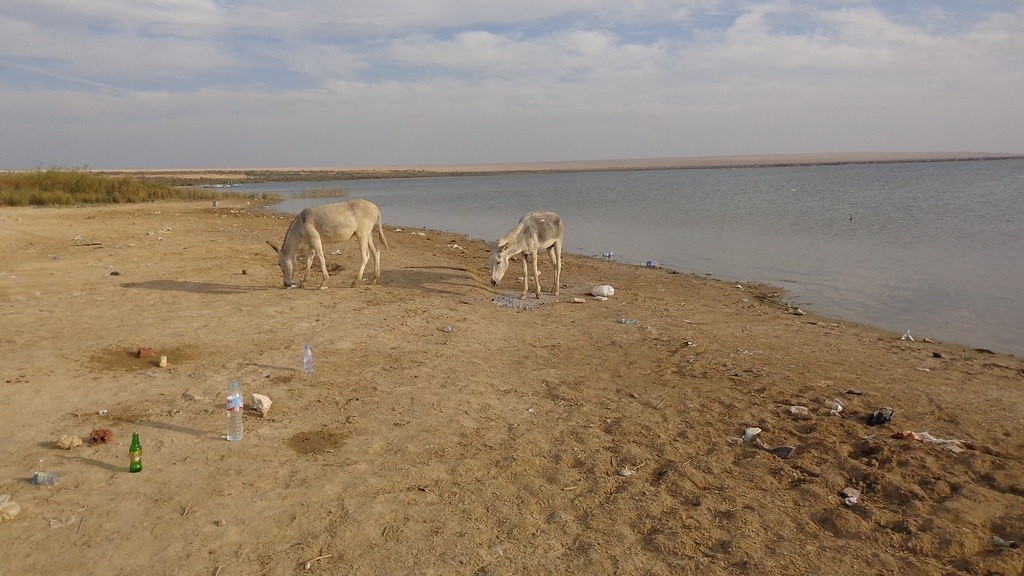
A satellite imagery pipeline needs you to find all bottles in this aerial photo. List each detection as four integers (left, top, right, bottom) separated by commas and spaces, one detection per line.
129, 432, 143, 473
303, 344, 313, 373
226, 382, 244, 441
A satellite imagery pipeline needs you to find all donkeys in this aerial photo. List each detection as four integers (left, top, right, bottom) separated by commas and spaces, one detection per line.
268, 199, 389, 289
492, 210, 564, 301
